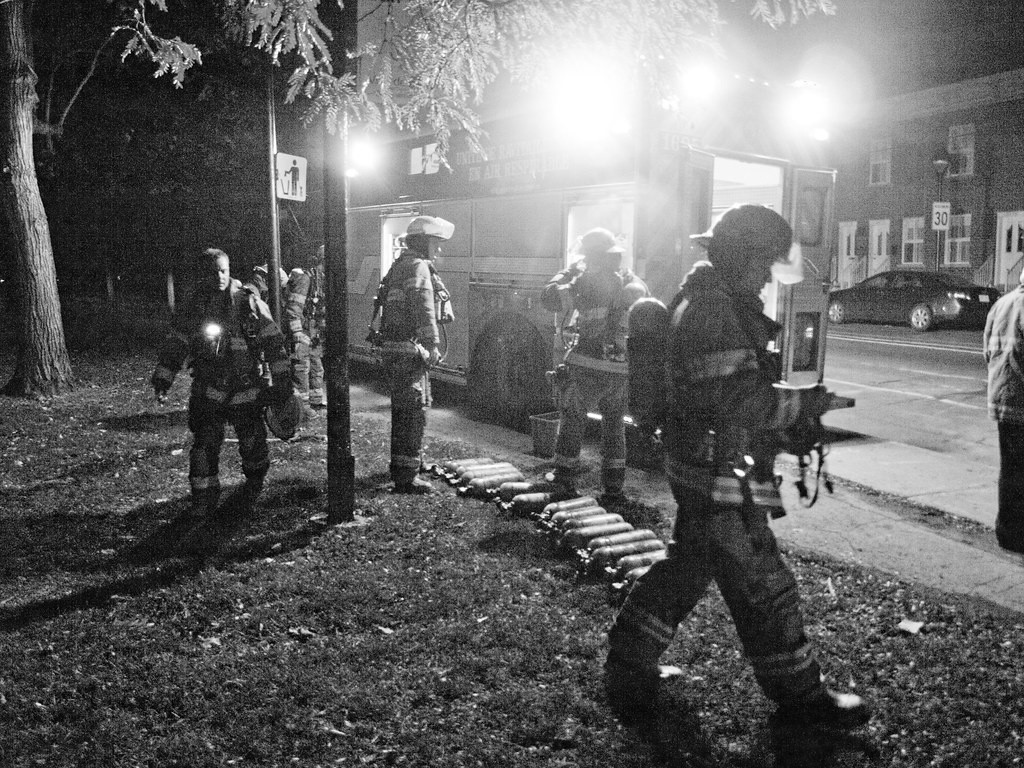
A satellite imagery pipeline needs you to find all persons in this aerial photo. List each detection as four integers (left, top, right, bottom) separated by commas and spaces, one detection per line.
542, 229, 651, 495
601, 203, 872, 729
983, 267, 1024, 552
378, 216, 456, 491
151, 245, 326, 517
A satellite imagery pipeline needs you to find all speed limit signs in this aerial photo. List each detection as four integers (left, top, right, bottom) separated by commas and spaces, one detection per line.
932, 202, 950, 233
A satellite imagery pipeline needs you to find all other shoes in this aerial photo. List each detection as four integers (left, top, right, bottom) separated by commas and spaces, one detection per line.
604, 492, 628, 503
310, 403, 325, 410
387, 471, 433, 494
543, 469, 572, 488
188, 494, 220, 517
776, 678, 872, 727
604, 649, 683, 689
245, 473, 264, 496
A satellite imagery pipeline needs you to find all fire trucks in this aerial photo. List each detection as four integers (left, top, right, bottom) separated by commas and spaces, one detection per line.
344, 143, 856, 436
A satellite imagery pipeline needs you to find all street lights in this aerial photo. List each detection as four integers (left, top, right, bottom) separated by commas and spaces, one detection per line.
928, 147, 954, 267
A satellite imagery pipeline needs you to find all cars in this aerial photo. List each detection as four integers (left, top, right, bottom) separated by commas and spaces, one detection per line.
824, 270, 1000, 331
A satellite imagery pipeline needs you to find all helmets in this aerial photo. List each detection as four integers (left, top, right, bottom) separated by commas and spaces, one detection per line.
689, 204, 795, 267
315, 245, 325, 261
578, 230, 623, 254
252, 263, 269, 274
265, 389, 304, 440
405, 216, 450, 241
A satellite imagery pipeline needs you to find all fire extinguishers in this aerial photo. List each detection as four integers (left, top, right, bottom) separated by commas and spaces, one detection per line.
419, 456, 667, 596
